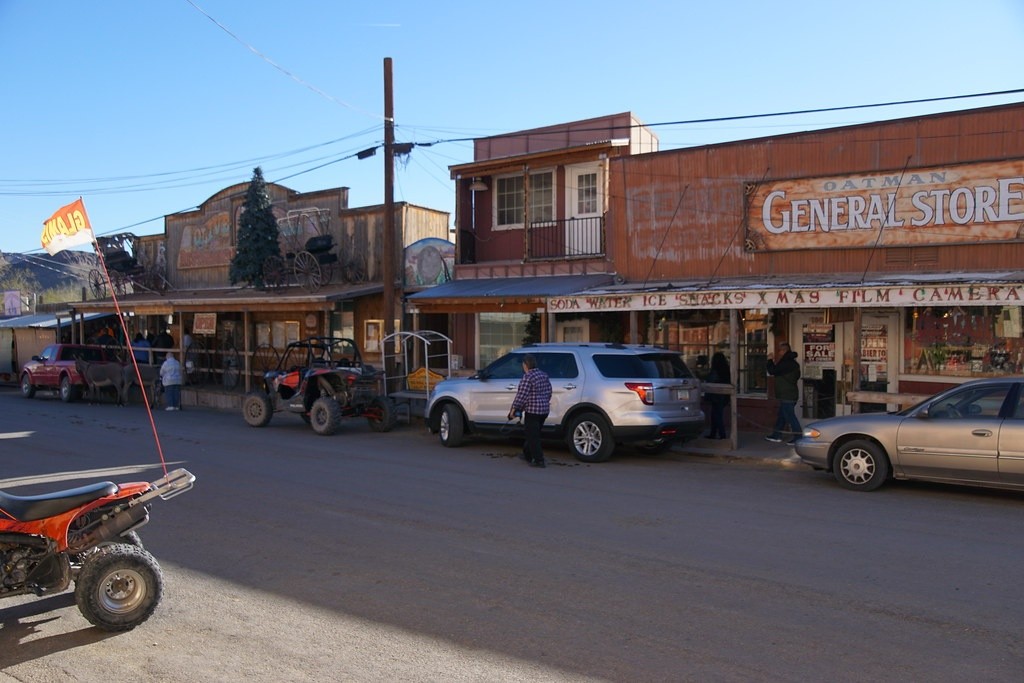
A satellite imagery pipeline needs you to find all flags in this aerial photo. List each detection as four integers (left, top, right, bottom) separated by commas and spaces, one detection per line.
41, 199, 94, 256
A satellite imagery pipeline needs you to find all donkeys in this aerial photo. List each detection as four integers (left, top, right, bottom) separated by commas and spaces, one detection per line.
72, 352, 196, 409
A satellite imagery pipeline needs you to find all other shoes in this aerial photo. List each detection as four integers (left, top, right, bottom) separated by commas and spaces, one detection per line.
529, 460, 544, 467
719, 437, 725, 440
518, 454, 531, 463
765, 436, 782, 443
786, 440, 795, 445
175, 407, 180, 411
165, 407, 174, 411
704, 435, 715, 439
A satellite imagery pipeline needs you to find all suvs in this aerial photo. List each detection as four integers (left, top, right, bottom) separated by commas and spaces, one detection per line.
424, 342, 706, 464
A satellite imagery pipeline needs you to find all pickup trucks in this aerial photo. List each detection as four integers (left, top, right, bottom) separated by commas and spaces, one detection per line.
19, 343, 130, 404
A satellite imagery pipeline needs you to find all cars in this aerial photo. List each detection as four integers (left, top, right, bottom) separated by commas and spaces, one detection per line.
244, 336, 398, 436
793, 376, 1024, 494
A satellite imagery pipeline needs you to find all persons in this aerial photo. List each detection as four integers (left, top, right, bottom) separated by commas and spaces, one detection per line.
97, 328, 112, 345
764, 342, 803, 445
510, 355, 552, 468
147, 327, 154, 342
184, 327, 192, 348
131, 332, 150, 363
152, 326, 174, 364
704, 352, 731, 439
160, 352, 183, 411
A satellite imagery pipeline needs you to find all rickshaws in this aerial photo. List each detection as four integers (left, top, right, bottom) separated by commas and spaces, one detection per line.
89, 231, 179, 301
261, 212, 368, 294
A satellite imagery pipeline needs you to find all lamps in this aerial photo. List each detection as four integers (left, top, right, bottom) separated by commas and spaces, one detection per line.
521, 258, 526, 265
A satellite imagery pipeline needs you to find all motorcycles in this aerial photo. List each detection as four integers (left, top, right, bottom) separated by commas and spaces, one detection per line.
0, 467, 197, 633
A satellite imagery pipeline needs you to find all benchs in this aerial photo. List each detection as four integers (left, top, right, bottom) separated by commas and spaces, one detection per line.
305, 234, 336, 261
105, 251, 134, 272
381, 332, 454, 428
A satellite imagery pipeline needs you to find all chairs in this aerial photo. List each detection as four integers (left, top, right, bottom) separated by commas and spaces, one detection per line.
339, 357, 351, 369
294, 357, 327, 397
